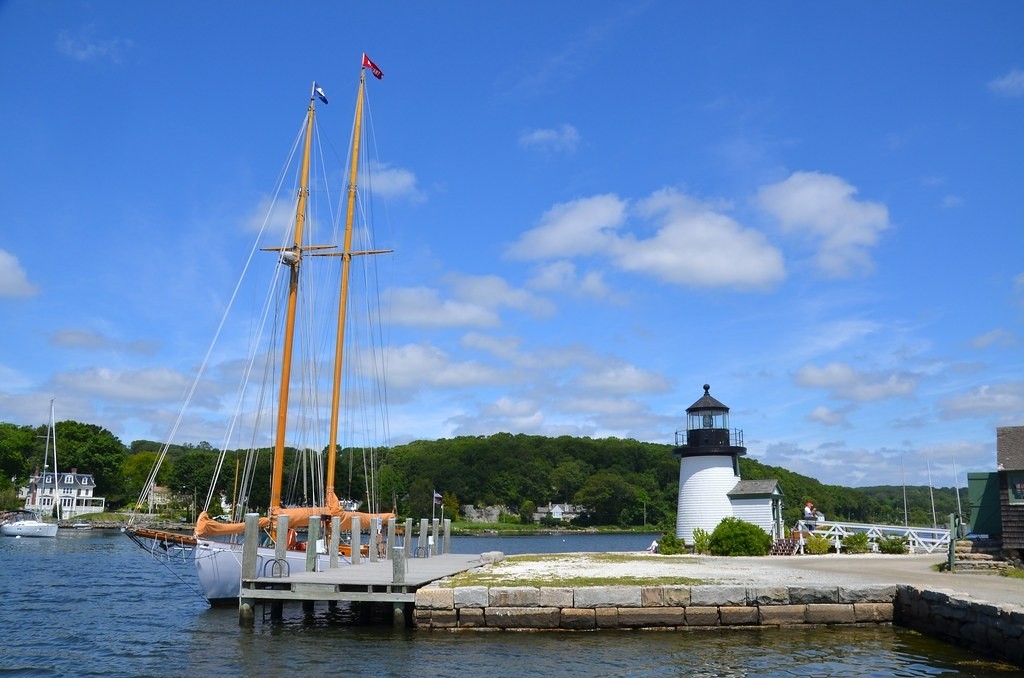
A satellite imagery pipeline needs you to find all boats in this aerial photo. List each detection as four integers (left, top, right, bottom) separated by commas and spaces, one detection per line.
72, 517, 94, 530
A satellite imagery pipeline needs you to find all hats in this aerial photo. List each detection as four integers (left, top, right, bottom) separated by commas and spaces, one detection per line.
807, 503, 813, 507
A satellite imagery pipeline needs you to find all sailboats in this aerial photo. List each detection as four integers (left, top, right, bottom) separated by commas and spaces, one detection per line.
107, 51, 432, 606
0, 397, 64, 538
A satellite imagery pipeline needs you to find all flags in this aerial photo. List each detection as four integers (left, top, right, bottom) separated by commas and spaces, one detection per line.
362, 53, 384, 80
433, 491, 442, 504
312, 80, 329, 104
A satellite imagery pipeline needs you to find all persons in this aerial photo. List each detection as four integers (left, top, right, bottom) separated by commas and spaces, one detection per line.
804, 502, 816, 517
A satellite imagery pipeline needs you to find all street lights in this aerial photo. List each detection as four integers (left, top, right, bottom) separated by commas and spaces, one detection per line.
182, 485, 197, 524
